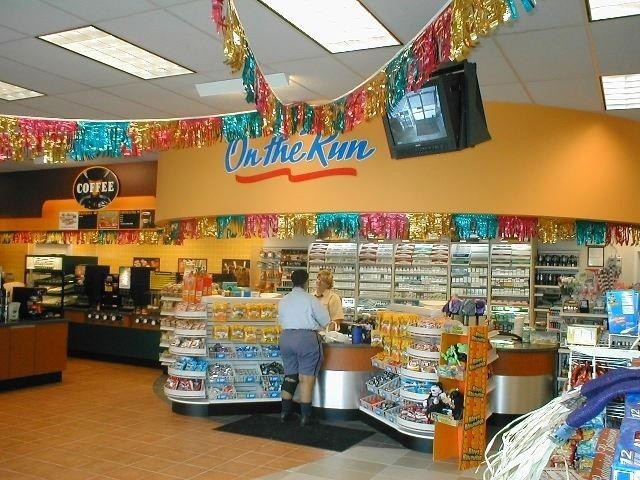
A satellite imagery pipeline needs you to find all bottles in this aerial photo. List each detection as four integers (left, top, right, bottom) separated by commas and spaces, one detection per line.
493, 312, 516, 332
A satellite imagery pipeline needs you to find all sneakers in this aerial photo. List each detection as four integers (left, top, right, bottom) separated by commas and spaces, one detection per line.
300, 417, 319, 428
280, 412, 302, 423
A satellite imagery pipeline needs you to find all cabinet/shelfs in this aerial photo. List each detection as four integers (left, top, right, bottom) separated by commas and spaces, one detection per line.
24, 268, 76, 307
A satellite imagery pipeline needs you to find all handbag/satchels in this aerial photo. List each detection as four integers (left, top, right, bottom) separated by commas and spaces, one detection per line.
324, 321, 351, 344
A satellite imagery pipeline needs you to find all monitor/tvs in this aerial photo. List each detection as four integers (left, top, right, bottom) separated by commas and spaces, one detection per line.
380, 69, 493, 160
13, 287, 48, 320
84, 265, 110, 287
119, 265, 132, 292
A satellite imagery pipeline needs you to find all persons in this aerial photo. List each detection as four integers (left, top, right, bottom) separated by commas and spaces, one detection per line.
310, 268, 345, 333
276, 268, 330, 429
